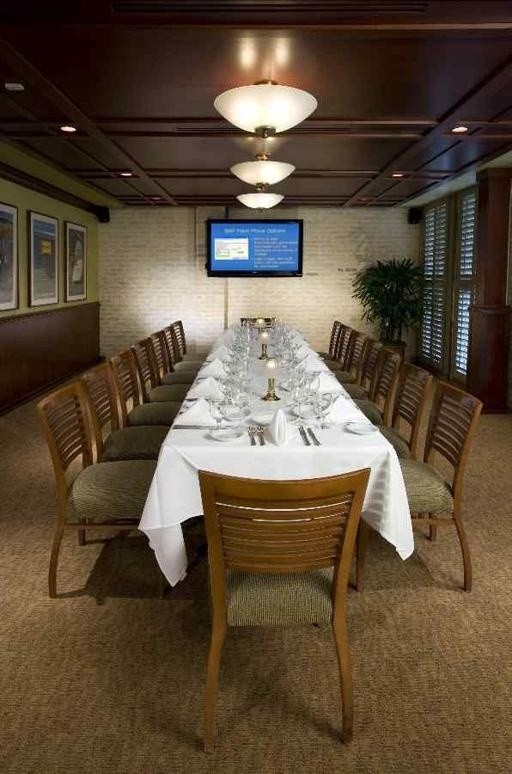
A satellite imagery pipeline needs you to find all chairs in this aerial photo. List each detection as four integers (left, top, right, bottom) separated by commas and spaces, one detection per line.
197, 466, 372, 755
239, 317, 277, 329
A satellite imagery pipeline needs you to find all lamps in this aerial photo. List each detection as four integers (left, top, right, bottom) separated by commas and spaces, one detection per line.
229, 153, 297, 190
235, 185, 286, 210
212, 78, 319, 139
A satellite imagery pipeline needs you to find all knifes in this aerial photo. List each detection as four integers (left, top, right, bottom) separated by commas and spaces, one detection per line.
298, 425, 321, 445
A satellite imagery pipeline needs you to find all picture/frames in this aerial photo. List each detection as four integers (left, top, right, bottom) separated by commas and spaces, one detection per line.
26, 210, 60, 307
0, 201, 19, 312
64, 220, 88, 303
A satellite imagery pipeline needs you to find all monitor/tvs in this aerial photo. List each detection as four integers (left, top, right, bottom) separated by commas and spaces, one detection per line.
206, 218, 304, 276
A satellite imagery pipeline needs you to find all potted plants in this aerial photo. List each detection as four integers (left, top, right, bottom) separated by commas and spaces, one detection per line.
351, 257, 432, 362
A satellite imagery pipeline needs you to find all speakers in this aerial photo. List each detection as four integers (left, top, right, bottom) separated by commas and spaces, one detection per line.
407, 207, 423, 224
97, 206, 110, 222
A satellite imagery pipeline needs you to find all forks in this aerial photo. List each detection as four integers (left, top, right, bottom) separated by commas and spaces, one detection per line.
248, 425, 264, 445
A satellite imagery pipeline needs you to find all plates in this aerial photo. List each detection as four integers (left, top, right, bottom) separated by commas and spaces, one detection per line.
211, 318, 379, 442
